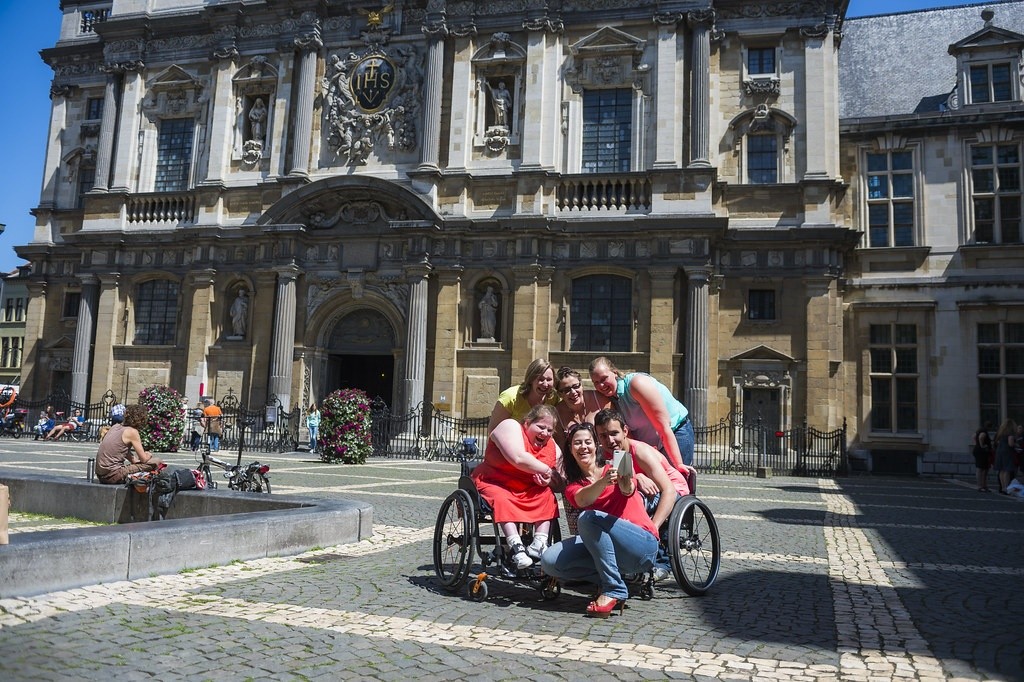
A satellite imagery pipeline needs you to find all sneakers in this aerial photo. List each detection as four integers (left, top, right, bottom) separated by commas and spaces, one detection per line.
506, 535, 533, 569
527, 533, 548, 558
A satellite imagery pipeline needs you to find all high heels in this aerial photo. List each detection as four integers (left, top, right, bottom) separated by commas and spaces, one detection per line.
586, 593, 626, 618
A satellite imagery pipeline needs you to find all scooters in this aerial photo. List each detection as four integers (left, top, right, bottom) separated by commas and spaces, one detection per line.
0, 406, 29, 439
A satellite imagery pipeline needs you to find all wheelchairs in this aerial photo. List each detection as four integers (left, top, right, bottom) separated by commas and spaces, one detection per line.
432, 452, 563, 602
620, 473, 722, 601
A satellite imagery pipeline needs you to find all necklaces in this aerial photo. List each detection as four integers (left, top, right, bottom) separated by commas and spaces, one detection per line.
570, 401, 586, 424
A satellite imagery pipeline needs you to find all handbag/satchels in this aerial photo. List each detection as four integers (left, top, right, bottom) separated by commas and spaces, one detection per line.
123, 463, 196, 521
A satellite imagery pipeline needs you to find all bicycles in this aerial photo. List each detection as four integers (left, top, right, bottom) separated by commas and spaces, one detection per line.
194, 441, 273, 494
426, 417, 479, 463
55, 412, 93, 443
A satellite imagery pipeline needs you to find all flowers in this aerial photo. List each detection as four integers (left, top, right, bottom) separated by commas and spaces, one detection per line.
315, 388, 375, 466
137, 384, 187, 451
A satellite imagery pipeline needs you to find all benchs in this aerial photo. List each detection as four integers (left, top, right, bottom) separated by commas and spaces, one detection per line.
31, 419, 93, 443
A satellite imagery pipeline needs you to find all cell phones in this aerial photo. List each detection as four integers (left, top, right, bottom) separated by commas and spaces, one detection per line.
613, 450, 626, 475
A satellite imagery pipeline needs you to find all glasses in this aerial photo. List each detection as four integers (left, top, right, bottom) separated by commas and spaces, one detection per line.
568, 422, 595, 437
558, 380, 582, 394
75, 412, 80, 413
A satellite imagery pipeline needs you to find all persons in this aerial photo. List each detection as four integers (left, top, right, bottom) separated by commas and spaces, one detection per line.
486, 80, 512, 126
95, 403, 161, 484
180, 397, 223, 452
32, 406, 56, 440
307, 403, 321, 453
326, 44, 421, 163
249, 98, 268, 140
541, 421, 660, 618
469, 356, 694, 579
972, 418, 1024, 495
110, 402, 126, 425
44, 409, 85, 442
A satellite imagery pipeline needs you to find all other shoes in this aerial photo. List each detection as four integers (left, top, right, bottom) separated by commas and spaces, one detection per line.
642, 566, 669, 583
52, 437, 58, 442
43, 437, 50, 441
